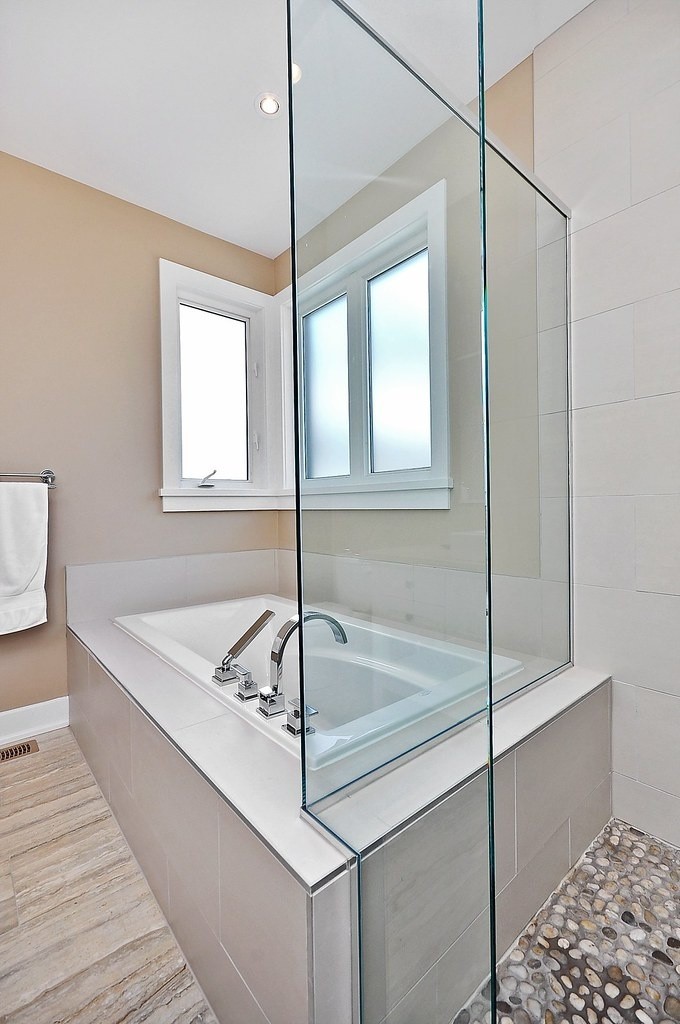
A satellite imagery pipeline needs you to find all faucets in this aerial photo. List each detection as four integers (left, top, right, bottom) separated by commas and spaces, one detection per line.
267, 612, 352, 688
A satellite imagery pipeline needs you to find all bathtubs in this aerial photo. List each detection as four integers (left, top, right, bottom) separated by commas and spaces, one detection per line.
67, 594, 614, 1023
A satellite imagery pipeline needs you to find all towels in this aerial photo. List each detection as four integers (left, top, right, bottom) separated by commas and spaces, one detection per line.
0, 484, 49, 634
